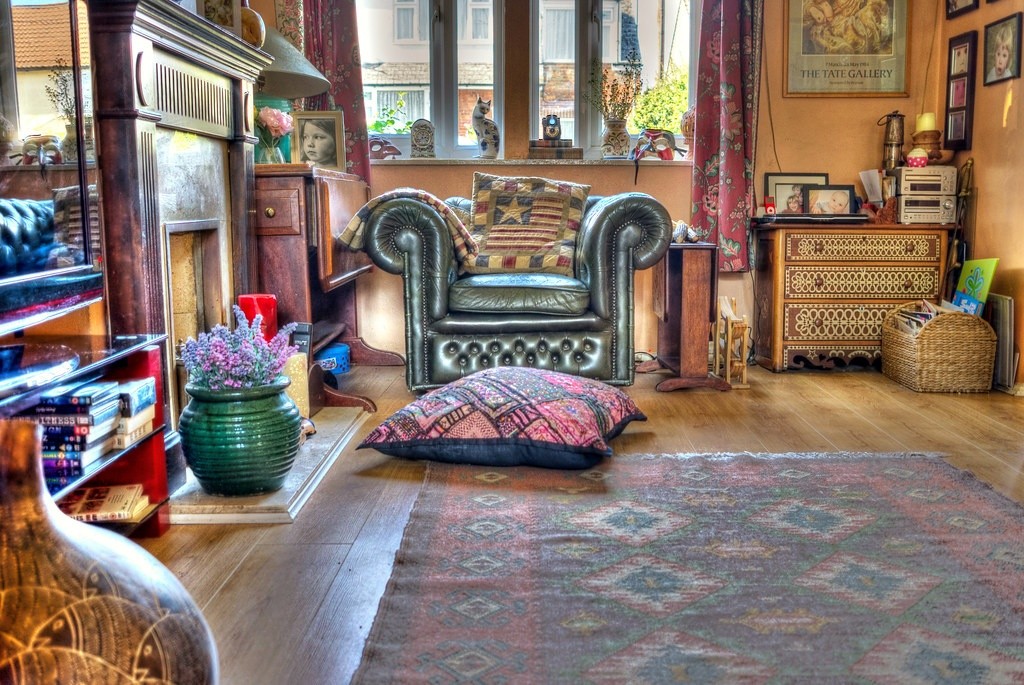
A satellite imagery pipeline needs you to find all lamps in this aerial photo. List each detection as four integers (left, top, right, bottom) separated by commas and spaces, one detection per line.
251, 24, 331, 163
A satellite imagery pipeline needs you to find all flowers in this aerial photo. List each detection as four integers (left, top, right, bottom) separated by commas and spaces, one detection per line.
253, 105, 296, 163
179, 304, 302, 392
583, 58, 644, 120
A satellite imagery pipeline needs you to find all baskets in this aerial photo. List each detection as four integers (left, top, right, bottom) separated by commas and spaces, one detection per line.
880, 301, 997, 394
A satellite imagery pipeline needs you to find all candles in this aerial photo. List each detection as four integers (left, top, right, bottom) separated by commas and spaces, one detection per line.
914, 113, 936, 131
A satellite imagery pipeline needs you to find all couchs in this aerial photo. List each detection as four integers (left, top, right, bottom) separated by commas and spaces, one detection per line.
365, 191, 672, 398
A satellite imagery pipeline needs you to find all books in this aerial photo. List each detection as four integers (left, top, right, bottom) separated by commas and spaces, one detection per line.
530, 139, 584, 159
12, 375, 157, 524
883, 176, 896, 200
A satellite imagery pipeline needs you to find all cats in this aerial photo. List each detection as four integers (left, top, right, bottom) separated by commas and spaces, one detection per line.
472, 97, 500, 159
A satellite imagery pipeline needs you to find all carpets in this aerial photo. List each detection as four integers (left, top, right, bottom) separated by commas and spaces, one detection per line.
351, 449, 1024, 685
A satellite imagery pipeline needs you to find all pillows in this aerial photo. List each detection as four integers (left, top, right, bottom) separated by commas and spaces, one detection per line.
355, 367, 649, 471
470, 171, 592, 278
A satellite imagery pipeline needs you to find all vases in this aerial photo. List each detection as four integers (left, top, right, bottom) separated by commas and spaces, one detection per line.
259, 147, 287, 163
600, 118, 630, 158
0, 419, 219, 685
178, 378, 301, 497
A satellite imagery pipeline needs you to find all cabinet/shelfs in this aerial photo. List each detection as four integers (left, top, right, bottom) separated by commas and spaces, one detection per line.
752, 225, 956, 373
0, 333, 172, 545
251, 161, 405, 415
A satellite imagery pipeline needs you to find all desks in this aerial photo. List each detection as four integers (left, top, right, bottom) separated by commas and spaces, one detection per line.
638, 240, 732, 393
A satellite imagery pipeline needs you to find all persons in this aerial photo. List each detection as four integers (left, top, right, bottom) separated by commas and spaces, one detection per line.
987, 23, 1013, 81
301, 119, 337, 166
953, 46, 967, 139
782, 185, 804, 213
811, 191, 848, 214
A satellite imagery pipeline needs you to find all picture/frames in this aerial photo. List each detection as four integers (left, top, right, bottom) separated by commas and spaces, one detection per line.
290, 110, 347, 171
782, 0, 1022, 150
765, 172, 857, 216
